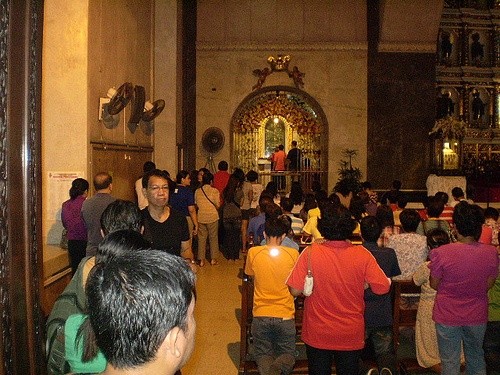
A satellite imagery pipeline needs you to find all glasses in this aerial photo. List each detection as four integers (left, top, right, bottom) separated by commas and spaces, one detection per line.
146, 184, 169, 192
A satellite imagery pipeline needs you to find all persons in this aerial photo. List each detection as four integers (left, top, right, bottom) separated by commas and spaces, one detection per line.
427, 201, 500, 375
413, 229, 468, 375
139, 169, 197, 274
484, 231, 499, 375
286, 66, 305, 89
267, 141, 321, 191
471, 31, 487, 61
441, 93, 457, 119
471, 91, 488, 124
135, 162, 264, 265
441, 31, 453, 64
61, 178, 90, 278
248, 178, 500, 251
351, 217, 401, 375
82, 172, 119, 256
85, 251, 197, 374
252, 67, 273, 91
47, 200, 145, 375
64, 229, 156, 374
286, 204, 391, 375
387, 209, 430, 280
245, 217, 299, 375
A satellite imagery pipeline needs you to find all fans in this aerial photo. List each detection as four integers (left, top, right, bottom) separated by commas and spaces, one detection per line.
99, 82, 165, 123
202, 127, 225, 174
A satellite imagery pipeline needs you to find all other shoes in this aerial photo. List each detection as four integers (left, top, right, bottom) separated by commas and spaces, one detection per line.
228, 256, 232, 261
380, 365, 392, 375
185, 258, 195, 265
198, 259, 206, 267
242, 249, 246, 253
365, 366, 379, 375
211, 258, 219, 265
234, 256, 239, 261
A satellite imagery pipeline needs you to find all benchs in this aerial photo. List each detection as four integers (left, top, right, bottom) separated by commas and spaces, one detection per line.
240, 208, 500, 375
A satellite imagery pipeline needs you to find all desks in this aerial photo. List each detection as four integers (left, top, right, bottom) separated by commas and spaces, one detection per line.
426, 174, 467, 203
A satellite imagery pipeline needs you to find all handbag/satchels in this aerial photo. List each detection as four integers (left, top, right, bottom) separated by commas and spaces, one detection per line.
303, 245, 314, 296
215, 206, 223, 221
59, 229, 69, 250
